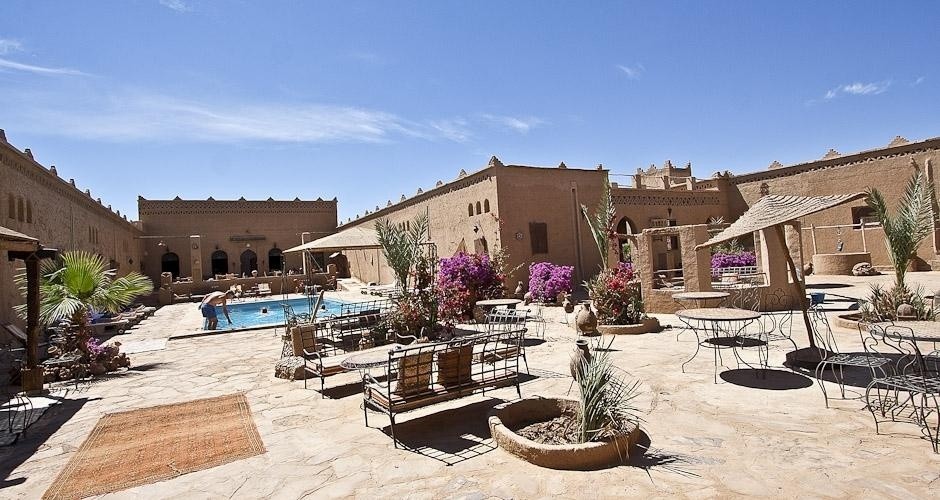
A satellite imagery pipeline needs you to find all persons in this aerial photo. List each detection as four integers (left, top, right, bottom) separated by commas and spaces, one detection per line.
198, 290, 234, 330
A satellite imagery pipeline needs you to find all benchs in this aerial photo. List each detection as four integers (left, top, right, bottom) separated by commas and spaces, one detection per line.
290, 294, 547, 401
361, 327, 528, 450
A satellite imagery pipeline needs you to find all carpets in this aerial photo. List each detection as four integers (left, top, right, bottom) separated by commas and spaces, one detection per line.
41, 387, 270, 500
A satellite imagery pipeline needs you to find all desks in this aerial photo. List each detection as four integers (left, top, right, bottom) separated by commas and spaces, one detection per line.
670, 289, 731, 340
675, 307, 761, 387
868, 320, 940, 393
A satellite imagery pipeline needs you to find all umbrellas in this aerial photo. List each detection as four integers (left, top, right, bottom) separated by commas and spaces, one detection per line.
695, 192, 868, 369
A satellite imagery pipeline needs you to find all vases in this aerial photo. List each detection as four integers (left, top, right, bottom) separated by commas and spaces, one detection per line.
573, 302, 597, 335
569, 337, 596, 383
513, 279, 575, 312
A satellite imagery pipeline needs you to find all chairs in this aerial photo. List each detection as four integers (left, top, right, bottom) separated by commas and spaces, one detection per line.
735, 288, 799, 378
807, 303, 899, 417
857, 318, 940, 454
